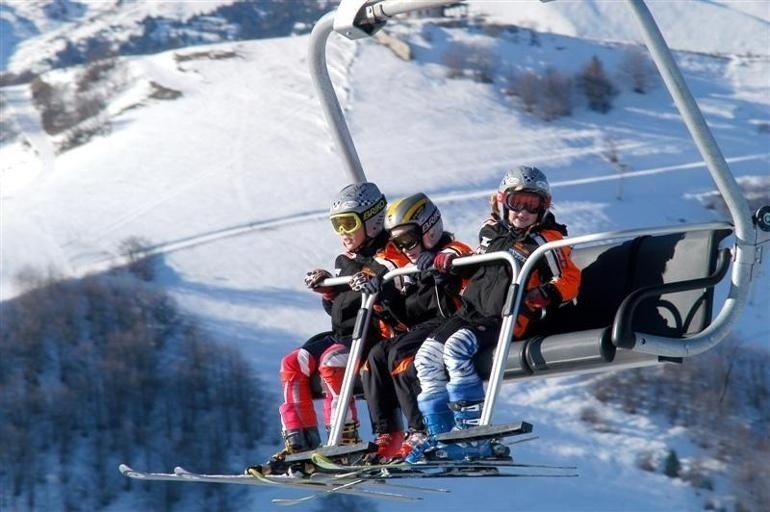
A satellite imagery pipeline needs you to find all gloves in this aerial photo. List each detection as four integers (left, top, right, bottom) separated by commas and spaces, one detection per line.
304, 269, 340, 301
414, 246, 463, 273
523, 281, 562, 312
347, 272, 385, 296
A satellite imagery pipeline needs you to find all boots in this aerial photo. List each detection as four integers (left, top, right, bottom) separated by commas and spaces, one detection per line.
244, 379, 493, 475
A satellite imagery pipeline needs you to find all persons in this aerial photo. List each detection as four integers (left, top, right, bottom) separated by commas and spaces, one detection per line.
361, 191, 475, 466
404, 165, 581, 472
245, 182, 391, 482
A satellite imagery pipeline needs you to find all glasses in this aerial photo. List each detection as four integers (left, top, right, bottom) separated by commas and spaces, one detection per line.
497, 190, 551, 214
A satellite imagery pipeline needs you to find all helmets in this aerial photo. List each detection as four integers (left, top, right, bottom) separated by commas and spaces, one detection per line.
497, 165, 552, 223
330, 182, 443, 250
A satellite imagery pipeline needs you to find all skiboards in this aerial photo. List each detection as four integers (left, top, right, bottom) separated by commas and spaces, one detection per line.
119, 436, 581, 507
